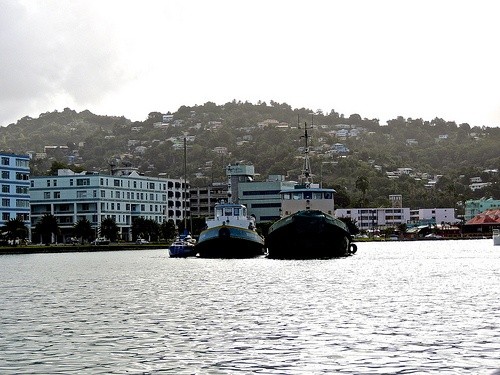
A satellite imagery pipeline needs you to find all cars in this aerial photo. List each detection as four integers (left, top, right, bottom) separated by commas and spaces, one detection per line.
351, 226, 445, 239
136, 239, 149, 245
92, 238, 110, 245
66, 237, 79, 244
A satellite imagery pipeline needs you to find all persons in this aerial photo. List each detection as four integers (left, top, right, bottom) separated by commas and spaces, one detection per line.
292, 194, 301, 200
252, 226, 257, 231
325, 193, 331, 199
248, 222, 254, 231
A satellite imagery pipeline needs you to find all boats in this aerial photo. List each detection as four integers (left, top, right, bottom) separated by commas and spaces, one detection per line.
195, 164, 264, 258
167, 230, 197, 257
265, 109, 357, 260
